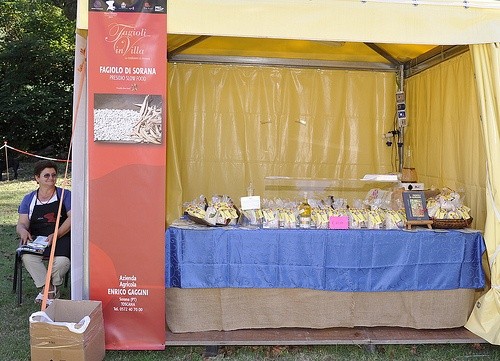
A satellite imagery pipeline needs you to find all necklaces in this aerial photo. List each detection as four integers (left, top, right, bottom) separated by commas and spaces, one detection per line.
37, 188, 56, 204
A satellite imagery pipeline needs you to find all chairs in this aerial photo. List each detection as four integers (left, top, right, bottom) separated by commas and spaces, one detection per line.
12, 251, 69, 304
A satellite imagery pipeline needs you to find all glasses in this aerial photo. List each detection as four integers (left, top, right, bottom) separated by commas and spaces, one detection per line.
40, 173, 57, 178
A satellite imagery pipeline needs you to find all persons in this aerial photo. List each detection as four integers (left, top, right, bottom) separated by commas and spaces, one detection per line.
16, 160, 71, 308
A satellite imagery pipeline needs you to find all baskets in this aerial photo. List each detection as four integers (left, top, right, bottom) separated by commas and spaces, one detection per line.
424, 215, 473, 229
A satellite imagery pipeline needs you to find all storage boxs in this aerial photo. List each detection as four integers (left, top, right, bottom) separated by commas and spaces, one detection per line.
30, 299, 106, 361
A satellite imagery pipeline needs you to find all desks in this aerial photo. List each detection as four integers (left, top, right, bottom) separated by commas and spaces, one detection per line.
165, 219, 487, 329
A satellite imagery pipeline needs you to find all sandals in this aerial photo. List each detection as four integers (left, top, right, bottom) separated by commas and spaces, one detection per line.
34, 293, 43, 304
46, 285, 59, 307
